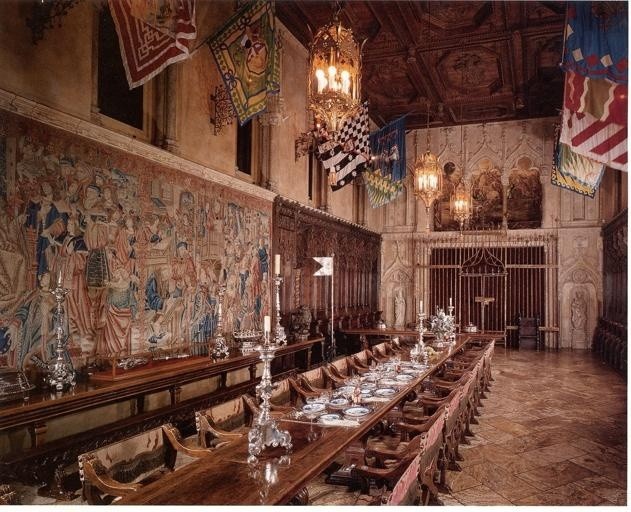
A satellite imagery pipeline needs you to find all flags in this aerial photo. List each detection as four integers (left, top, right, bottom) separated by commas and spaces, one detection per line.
107, 0, 197, 90
205, 0, 284, 127
318, 101, 372, 192
550, 3, 628, 199
361, 119, 406, 209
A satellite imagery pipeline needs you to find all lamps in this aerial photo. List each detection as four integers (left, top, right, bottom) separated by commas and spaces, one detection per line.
412, 0, 445, 216
305, 1, 367, 186
448, 1, 474, 231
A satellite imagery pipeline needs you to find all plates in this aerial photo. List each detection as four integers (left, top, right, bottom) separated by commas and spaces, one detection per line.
303, 360, 431, 421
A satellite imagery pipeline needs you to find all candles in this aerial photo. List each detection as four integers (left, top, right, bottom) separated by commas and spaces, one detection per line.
264, 314, 272, 342
449, 298, 454, 307
420, 300, 424, 314
273, 253, 282, 277
219, 303, 222, 314
